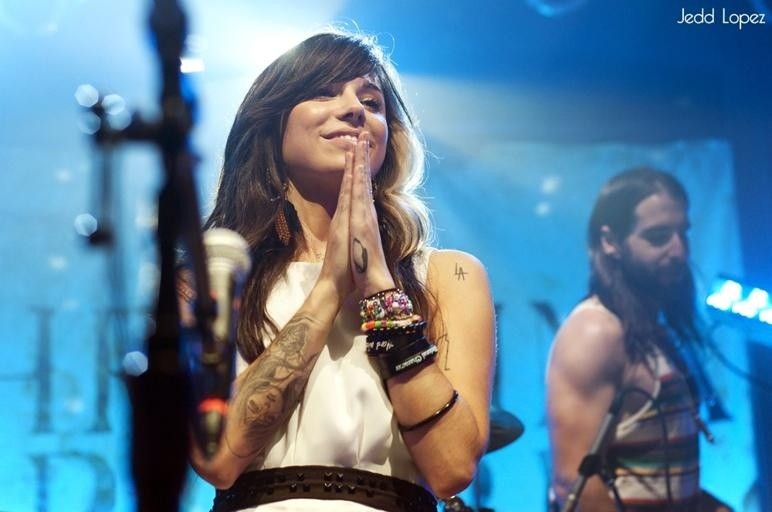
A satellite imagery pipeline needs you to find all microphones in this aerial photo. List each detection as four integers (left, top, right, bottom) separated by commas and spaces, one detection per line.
563, 398, 622, 512
195, 228, 252, 456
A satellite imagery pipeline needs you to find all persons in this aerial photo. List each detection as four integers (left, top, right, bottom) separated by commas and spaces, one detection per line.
172, 28, 496, 512
545, 164, 734, 512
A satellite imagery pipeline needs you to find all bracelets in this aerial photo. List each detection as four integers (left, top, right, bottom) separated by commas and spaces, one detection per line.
359, 286, 438, 382
397, 390, 458, 433
